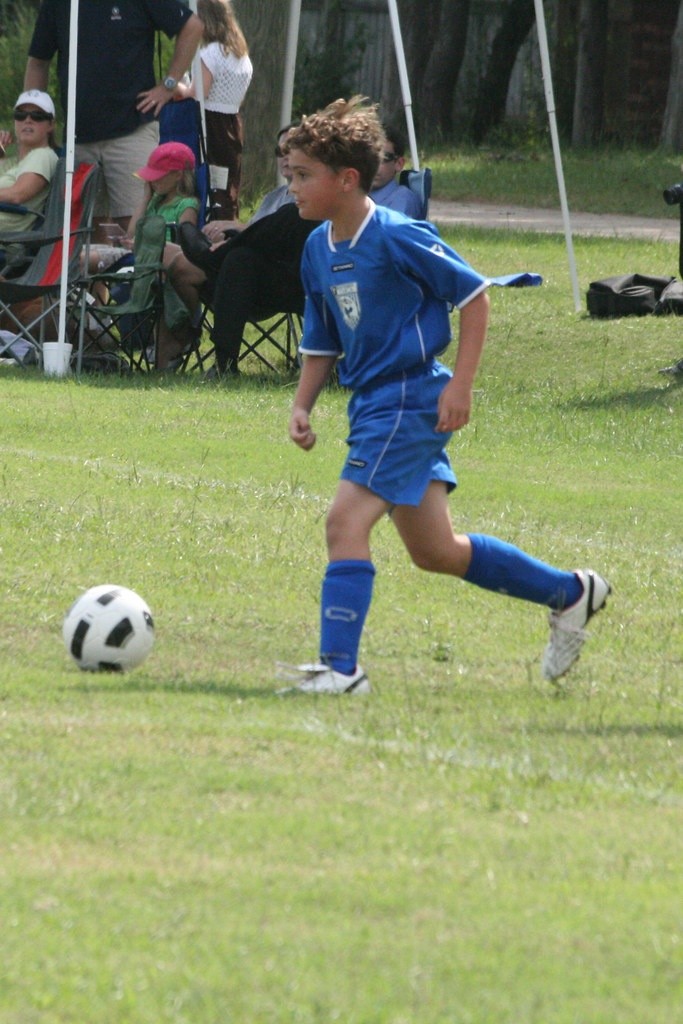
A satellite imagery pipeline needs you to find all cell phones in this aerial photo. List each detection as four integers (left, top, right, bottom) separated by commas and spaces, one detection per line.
100, 223, 128, 242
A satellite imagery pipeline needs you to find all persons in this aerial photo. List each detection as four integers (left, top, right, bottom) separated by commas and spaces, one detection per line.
155, 123, 420, 381
176, 0, 253, 220
278, 88, 612, 698
132, 143, 199, 360
25, 0, 205, 351
0, 90, 61, 273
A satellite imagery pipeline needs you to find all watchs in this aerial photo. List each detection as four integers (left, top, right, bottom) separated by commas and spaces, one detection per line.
162, 77, 178, 90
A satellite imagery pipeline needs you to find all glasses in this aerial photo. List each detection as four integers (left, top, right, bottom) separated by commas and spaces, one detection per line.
273, 144, 291, 158
382, 148, 399, 162
14, 109, 52, 122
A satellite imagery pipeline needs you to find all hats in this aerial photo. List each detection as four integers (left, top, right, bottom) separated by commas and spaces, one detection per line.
13, 88, 54, 117
138, 140, 198, 181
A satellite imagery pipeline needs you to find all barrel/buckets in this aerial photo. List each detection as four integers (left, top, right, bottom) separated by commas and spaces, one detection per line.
43, 342, 72, 376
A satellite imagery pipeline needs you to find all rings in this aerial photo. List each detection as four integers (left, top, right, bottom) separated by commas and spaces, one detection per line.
151, 101, 156, 105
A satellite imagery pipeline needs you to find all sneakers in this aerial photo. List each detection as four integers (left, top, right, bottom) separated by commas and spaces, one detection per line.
273, 660, 373, 697
542, 570, 610, 681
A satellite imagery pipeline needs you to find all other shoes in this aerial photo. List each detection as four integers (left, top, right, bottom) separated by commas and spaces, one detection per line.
198, 360, 240, 381
180, 220, 215, 283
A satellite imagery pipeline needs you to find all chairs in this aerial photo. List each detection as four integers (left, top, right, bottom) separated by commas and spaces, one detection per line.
0, 155, 433, 376
